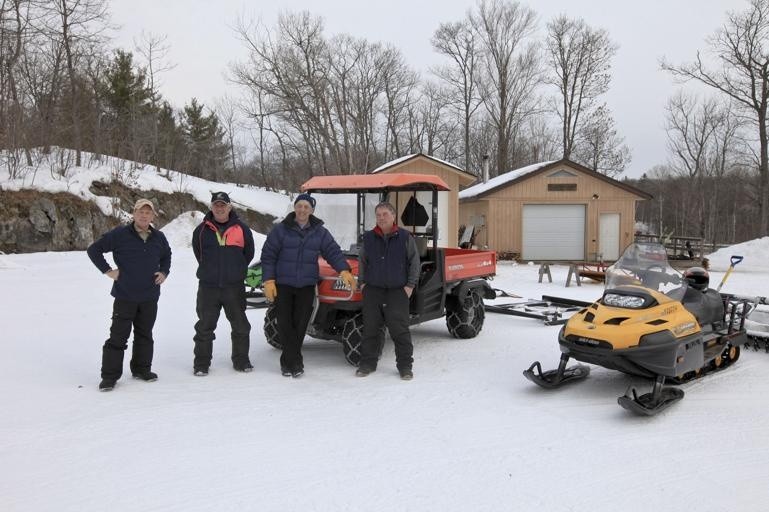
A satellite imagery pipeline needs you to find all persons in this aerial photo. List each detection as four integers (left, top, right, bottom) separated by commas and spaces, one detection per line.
356, 202, 422, 379
87, 198, 172, 391
192, 192, 254, 376
261, 194, 356, 378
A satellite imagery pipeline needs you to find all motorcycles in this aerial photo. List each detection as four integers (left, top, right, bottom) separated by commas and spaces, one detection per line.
523, 241, 756, 417
231, 259, 276, 310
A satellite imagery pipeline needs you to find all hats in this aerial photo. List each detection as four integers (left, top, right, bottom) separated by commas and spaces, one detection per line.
212, 191, 231, 204
294, 194, 316, 207
134, 199, 154, 209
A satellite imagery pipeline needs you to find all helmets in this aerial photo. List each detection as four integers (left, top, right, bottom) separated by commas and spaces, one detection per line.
684, 267, 711, 289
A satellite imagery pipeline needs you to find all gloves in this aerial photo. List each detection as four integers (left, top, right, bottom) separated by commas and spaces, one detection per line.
264, 280, 277, 302
341, 271, 357, 289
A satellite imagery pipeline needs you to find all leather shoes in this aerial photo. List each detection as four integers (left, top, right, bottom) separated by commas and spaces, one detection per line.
194, 365, 210, 376
282, 367, 291, 376
99, 378, 116, 390
356, 366, 376, 376
400, 367, 414, 380
293, 364, 304, 376
234, 361, 252, 372
134, 371, 156, 381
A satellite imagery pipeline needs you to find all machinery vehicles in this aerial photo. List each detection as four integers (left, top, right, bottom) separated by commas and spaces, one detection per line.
264, 173, 497, 366
